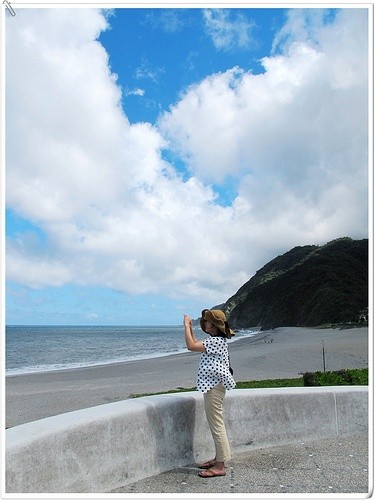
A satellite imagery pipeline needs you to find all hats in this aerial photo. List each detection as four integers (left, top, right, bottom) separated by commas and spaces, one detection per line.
200, 309, 235, 339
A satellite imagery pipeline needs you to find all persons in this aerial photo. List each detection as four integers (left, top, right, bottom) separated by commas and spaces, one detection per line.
183, 309, 238, 477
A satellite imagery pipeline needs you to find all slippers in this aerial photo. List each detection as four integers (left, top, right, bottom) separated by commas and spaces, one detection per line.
198, 460, 225, 477
